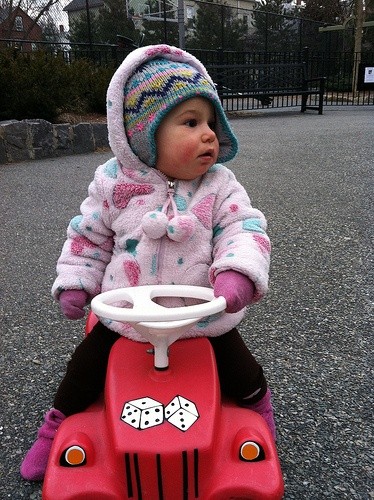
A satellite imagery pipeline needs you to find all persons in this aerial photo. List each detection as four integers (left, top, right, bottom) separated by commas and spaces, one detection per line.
20, 44, 276, 481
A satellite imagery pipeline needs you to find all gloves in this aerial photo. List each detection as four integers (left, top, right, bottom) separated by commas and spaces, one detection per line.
59, 290, 89, 320
214, 270, 255, 313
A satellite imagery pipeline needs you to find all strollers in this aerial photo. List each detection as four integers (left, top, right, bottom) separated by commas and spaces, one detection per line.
42, 284, 285, 500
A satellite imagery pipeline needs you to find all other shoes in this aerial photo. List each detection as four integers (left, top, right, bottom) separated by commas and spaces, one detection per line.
247, 388, 275, 441
20, 410, 67, 480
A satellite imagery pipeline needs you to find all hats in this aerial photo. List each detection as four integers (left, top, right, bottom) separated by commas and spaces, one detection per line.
123, 57, 238, 166
